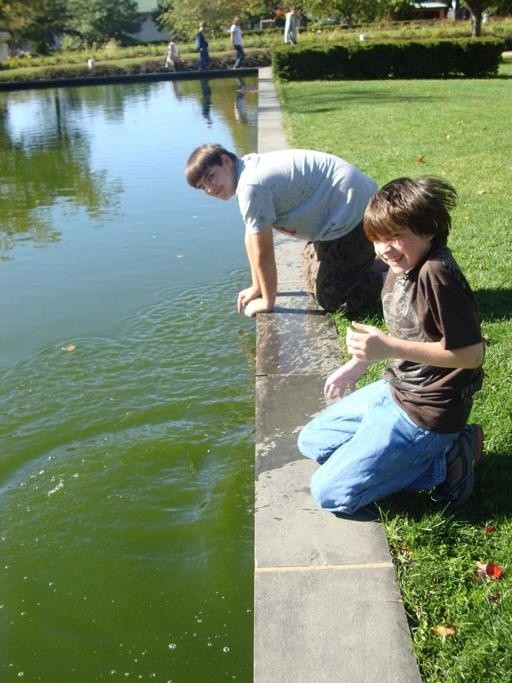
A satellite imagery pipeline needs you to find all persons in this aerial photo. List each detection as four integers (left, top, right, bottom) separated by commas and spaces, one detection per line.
195, 20, 210, 70
166, 77, 249, 130
188, 143, 385, 324
228, 15, 245, 68
297, 177, 487, 514
284, 6, 302, 47
166, 29, 179, 72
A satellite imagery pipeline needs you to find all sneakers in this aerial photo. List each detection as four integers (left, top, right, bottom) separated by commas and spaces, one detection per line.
438, 423, 485, 513
